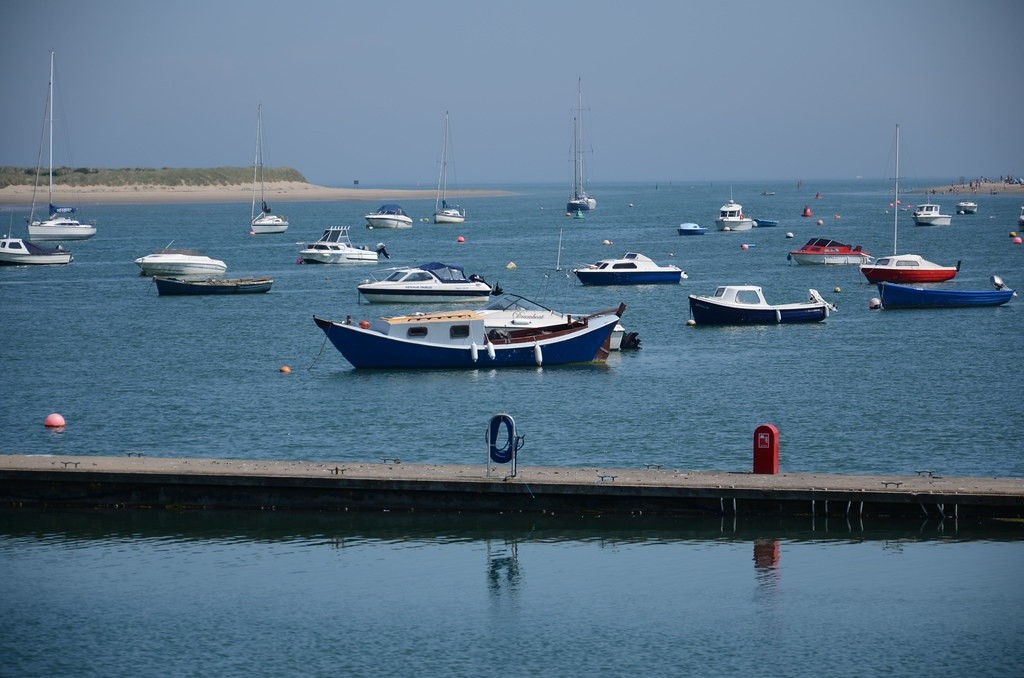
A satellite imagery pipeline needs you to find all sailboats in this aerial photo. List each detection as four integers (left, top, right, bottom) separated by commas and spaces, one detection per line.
566, 74, 598, 211
433, 110, 467, 224
252, 104, 290, 234
27, 51, 97, 242
860, 122, 962, 283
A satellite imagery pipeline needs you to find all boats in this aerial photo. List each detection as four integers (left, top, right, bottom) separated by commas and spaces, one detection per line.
1018, 206, 1024, 231
314, 292, 643, 370
574, 252, 688, 286
676, 223, 709, 235
792, 238, 872, 267
153, 276, 274, 295
956, 200, 978, 213
689, 284, 838, 328
135, 248, 230, 275
911, 203, 952, 225
0, 237, 74, 268
714, 187, 781, 232
298, 202, 413, 269
876, 275, 1014, 309
357, 259, 503, 301
